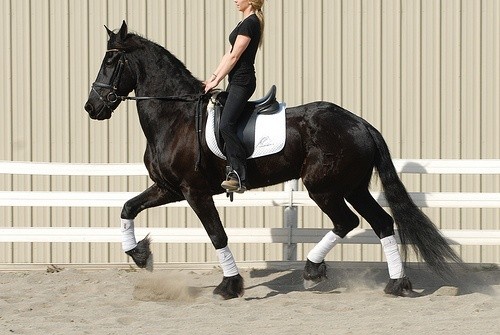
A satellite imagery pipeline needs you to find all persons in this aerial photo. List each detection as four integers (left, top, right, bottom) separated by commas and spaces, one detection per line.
201, 0, 265, 194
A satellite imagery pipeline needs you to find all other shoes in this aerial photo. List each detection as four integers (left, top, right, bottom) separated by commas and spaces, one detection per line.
220, 177, 247, 194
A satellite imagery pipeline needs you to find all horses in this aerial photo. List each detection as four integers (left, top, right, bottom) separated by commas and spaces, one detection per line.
84, 20, 462, 299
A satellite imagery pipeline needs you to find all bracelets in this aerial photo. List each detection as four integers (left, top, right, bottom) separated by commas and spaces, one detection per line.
213, 74, 217, 77
